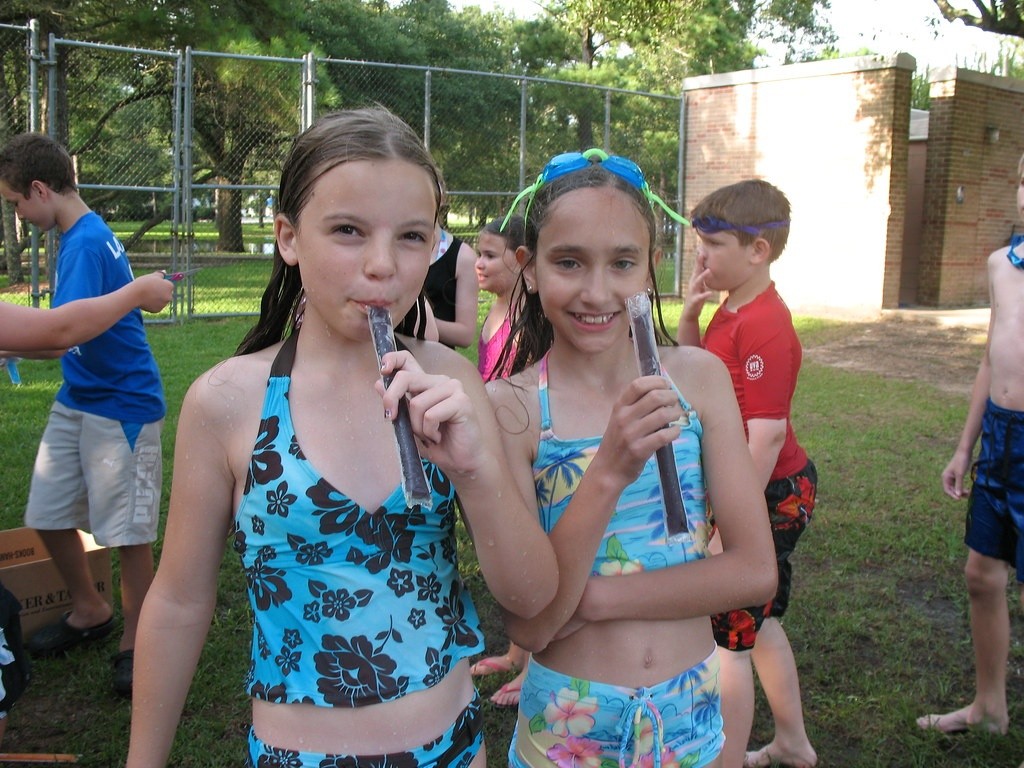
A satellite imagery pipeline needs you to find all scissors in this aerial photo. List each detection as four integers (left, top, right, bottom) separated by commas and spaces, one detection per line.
156, 267, 203, 282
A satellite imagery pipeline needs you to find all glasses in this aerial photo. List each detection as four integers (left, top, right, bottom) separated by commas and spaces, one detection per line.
500, 148, 691, 244
1006, 235, 1024, 270
692, 217, 789, 234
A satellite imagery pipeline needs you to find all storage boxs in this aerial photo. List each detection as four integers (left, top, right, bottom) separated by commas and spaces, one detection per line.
0, 527, 114, 644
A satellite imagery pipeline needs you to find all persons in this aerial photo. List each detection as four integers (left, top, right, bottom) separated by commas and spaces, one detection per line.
0, 132, 175, 709
126, 111, 818, 768
916, 153, 1024, 732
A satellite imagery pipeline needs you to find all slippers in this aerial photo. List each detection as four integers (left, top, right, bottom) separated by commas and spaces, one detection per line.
487, 682, 521, 709
112, 650, 134, 700
470, 656, 509, 675
30, 611, 114, 654
